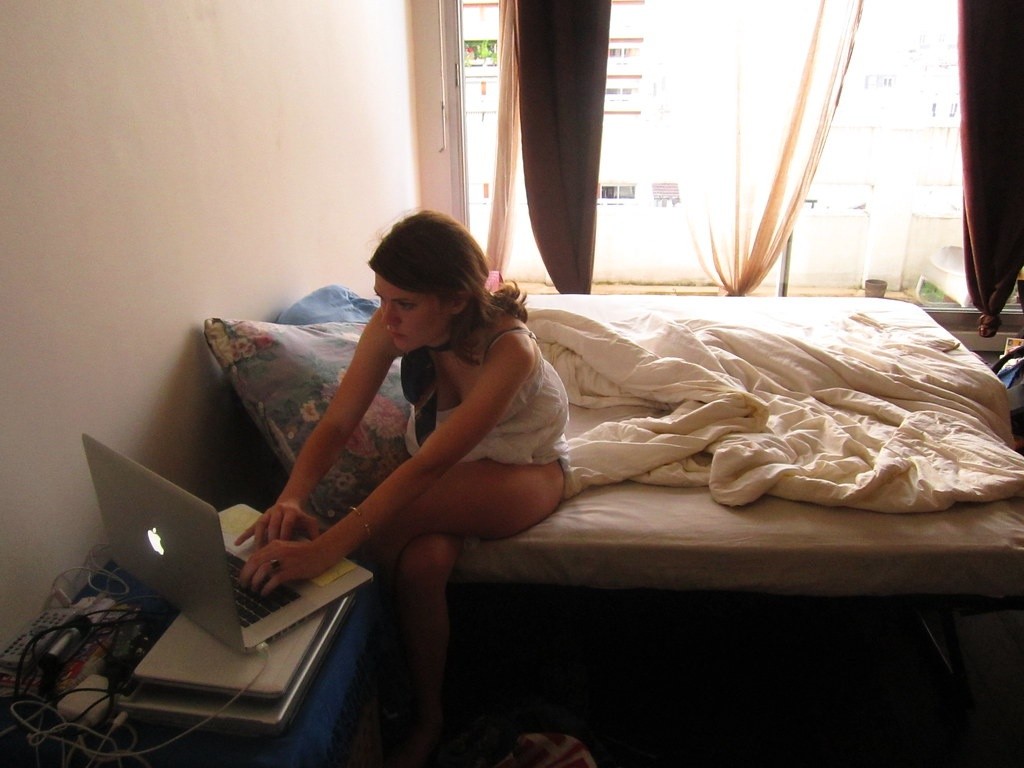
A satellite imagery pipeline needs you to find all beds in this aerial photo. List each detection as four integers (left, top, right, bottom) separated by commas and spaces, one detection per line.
255, 290, 1024, 592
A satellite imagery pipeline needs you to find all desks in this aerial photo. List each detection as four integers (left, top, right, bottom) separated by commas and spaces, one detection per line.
1, 563, 383, 768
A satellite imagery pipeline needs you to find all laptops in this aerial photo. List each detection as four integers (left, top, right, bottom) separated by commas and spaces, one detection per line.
132, 606, 333, 699
117, 589, 357, 739
81, 431, 373, 655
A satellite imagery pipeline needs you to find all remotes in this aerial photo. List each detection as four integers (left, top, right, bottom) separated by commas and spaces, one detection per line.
0, 606, 81, 668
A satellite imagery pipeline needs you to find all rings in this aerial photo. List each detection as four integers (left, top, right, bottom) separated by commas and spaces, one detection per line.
270, 559, 281, 571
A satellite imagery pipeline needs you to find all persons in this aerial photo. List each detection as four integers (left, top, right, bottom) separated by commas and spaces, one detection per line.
235, 211, 567, 768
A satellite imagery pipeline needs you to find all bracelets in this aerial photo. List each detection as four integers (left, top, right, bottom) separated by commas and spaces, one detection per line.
350, 506, 373, 539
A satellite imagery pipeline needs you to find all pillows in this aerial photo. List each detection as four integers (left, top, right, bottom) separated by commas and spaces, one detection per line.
202, 317, 411, 524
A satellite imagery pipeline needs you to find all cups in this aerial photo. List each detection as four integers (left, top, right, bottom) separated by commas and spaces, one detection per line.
865, 279, 888, 298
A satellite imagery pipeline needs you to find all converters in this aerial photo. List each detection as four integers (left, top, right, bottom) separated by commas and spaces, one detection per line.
106, 619, 146, 666
